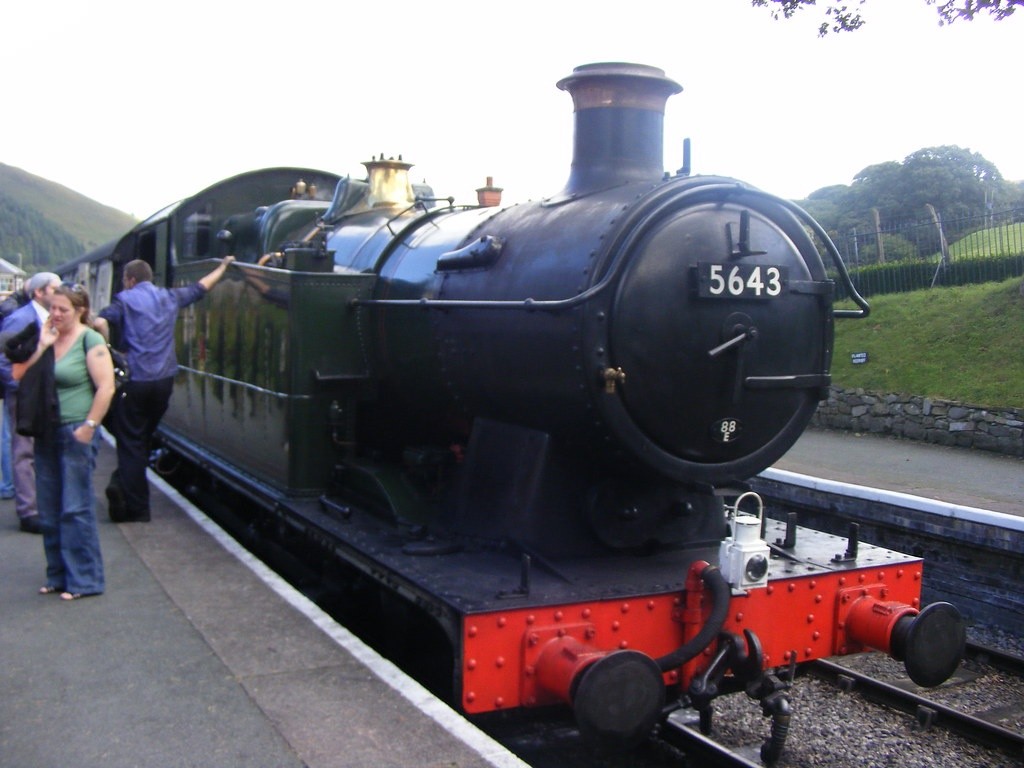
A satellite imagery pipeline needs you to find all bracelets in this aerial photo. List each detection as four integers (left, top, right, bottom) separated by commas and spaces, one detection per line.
85, 419, 97, 430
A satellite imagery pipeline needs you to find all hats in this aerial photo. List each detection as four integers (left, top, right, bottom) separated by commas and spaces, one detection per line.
29, 271, 57, 299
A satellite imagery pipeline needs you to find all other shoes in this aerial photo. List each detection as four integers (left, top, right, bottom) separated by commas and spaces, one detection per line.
40, 583, 57, 593
21, 515, 42, 534
61, 590, 83, 600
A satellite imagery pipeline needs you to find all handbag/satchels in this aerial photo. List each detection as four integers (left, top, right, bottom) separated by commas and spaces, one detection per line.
108, 343, 129, 391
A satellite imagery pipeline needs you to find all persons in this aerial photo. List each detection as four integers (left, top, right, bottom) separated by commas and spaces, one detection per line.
244, 270, 290, 306
0, 270, 116, 601
91, 256, 235, 522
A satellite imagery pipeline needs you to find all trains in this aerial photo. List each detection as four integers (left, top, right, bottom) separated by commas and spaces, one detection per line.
16, 62, 972, 768
